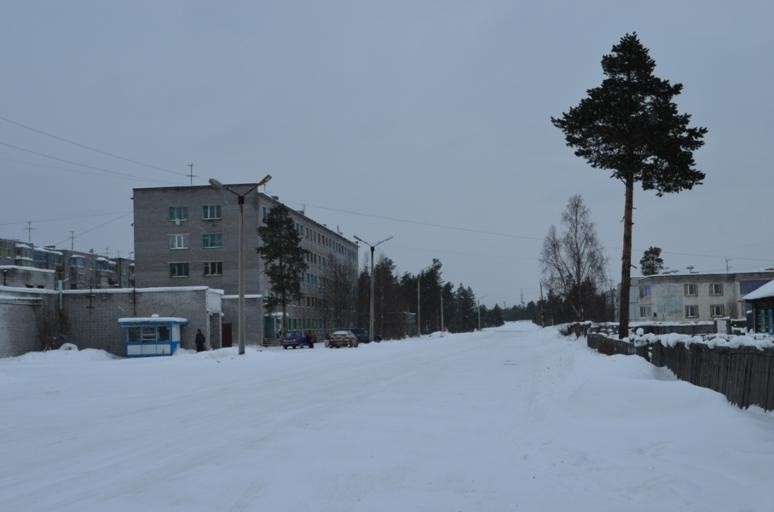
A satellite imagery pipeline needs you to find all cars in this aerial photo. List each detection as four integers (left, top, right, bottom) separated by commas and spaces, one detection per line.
325, 329, 359, 347
280, 328, 315, 348
352, 328, 369, 344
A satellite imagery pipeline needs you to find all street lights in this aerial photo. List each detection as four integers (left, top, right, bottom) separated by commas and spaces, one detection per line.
351, 235, 397, 340
209, 174, 271, 351
415, 261, 488, 335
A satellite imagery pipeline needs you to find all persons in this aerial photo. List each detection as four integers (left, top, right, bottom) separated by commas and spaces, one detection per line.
325, 332, 331, 340
307, 329, 315, 348
195, 329, 205, 352
